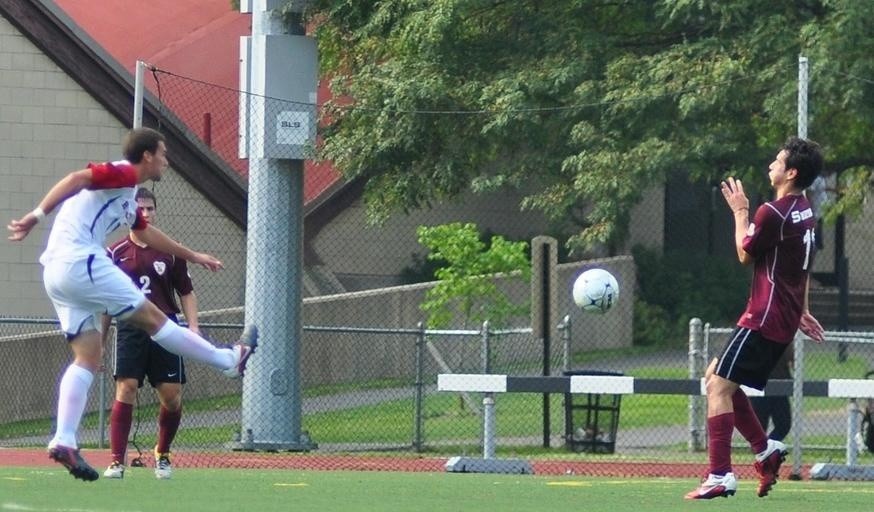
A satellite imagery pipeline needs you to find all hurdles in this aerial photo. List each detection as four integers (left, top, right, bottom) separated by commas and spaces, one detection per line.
435, 372, 874, 483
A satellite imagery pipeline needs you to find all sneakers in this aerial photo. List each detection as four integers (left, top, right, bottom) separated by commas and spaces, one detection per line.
47, 444, 124, 481
752, 438, 789, 497
153, 444, 170, 478
220, 320, 259, 380
684, 472, 737, 499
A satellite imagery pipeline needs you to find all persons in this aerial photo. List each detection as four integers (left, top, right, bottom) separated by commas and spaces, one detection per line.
6, 127, 258, 482
679, 137, 823, 500
99, 188, 204, 481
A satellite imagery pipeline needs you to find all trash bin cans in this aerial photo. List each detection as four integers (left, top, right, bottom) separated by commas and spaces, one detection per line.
561, 370, 624, 454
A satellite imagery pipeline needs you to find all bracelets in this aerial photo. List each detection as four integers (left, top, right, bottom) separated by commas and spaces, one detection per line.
733, 207, 748, 215
31, 207, 46, 222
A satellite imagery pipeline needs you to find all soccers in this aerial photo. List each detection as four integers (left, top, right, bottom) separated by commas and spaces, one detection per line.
573, 269, 619, 314
